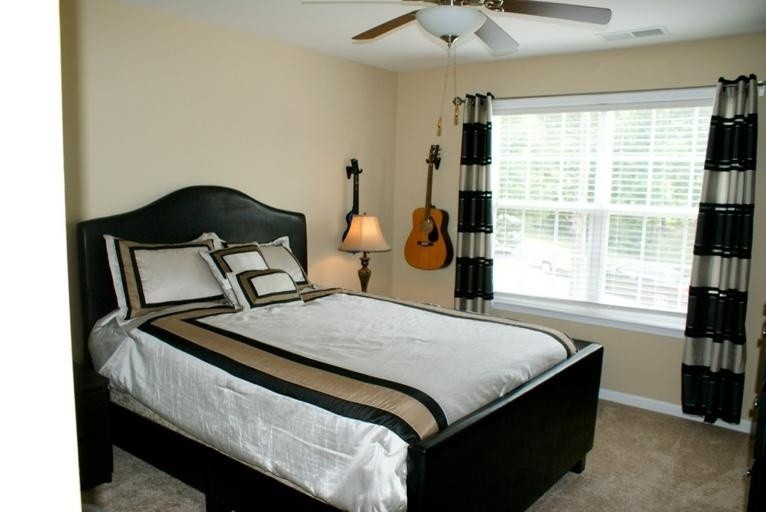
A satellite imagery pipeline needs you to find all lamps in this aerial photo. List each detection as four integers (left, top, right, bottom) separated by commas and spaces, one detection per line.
337, 212, 392, 293
415, 0, 487, 49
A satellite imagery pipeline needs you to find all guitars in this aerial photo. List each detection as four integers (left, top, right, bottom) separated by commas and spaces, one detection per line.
404, 144, 453, 271
342, 159, 363, 243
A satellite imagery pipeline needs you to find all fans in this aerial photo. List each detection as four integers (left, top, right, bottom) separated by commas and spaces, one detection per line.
352, 0, 611, 54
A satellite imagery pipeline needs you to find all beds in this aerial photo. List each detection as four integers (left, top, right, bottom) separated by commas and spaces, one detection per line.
77, 185, 604, 512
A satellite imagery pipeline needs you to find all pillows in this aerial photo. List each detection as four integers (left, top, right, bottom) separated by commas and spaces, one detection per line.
199, 241, 271, 310
206, 236, 315, 290
225, 269, 307, 313
103, 233, 234, 326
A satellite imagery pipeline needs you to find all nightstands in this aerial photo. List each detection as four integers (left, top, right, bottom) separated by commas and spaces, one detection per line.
73, 362, 114, 491
